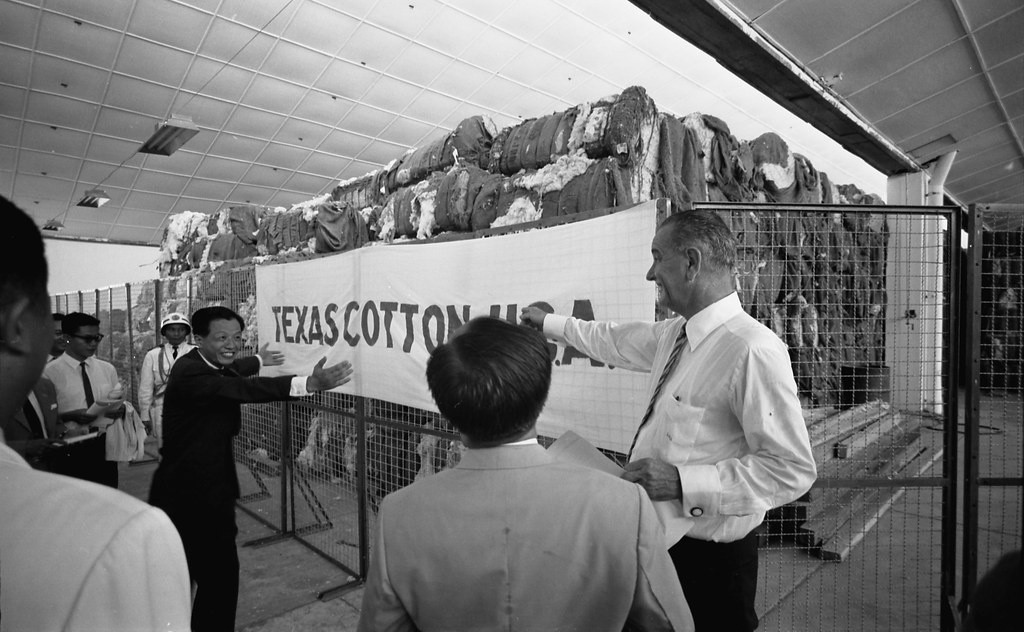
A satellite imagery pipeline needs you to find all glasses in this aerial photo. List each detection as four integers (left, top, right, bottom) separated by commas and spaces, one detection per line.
69, 334, 104, 343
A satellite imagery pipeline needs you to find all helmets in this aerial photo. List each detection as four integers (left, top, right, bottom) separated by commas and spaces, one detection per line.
161, 313, 191, 337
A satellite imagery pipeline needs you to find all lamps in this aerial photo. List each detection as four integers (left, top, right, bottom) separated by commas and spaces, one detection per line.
43, 219, 64, 231
76, 189, 110, 210
136, 112, 199, 157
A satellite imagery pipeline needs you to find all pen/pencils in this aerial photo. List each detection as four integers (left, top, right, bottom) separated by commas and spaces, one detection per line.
674, 395, 680, 401
57, 430, 69, 439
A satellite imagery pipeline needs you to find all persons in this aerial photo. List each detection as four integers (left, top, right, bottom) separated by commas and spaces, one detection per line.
0, 194, 191, 632
2, 377, 87, 476
138, 312, 199, 461
45, 313, 66, 364
520, 210, 818, 632
42, 312, 125, 489
147, 306, 354, 632
357, 316, 695, 632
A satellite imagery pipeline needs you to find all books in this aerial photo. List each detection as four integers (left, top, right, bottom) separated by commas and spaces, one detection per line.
86, 400, 124, 428
52, 431, 103, 446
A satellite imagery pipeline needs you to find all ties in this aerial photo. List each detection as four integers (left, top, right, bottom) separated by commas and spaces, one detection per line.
22, 398, 44, 440
173, 345, 178, 360
80, 362, 99, 433
626, 323, 687, 464
